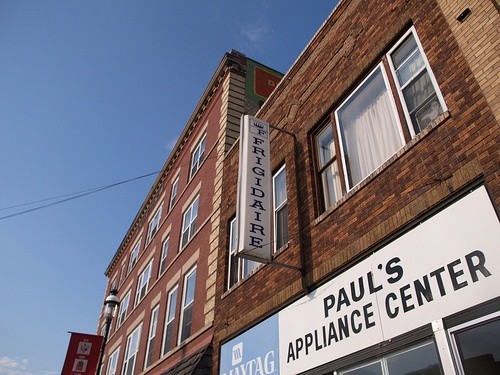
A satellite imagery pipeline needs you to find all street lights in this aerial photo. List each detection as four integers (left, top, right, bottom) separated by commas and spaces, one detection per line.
96, 286, 120, 375
